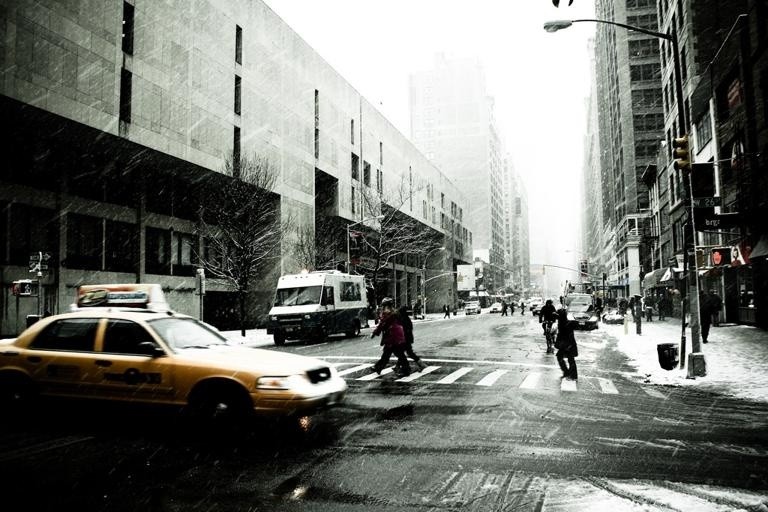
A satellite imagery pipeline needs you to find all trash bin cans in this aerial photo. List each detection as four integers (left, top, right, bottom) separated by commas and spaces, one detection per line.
657, 343, 680, 371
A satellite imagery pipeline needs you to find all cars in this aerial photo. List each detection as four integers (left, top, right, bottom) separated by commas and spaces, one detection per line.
518, 300, 526, 307
490, 302, 502, 313
566, 298, 598, 330
530, 300, 541, 310
464, 304, 481, 316
533, 304, 545, 316
1, 282, 349, 433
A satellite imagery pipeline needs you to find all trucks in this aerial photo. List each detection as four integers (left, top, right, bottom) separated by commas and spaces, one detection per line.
267, 268, 368, 345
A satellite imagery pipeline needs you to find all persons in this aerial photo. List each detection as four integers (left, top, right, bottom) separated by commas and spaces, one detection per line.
369, 296, 427, 376
521, 302, 524, 314
501, 301, 505, 311
510, 302, 515, 314
591, 289, 722, 343
443, 301, 465, 319
502, 303, 507, 316
539, 299, 556, 335
556, 308, 579, 379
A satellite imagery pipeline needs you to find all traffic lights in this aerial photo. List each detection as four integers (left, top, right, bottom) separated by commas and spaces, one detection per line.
672, 136, 691, 174
711, 249, 731, 265
603, 273, 607, 281
19, 282, 31, 295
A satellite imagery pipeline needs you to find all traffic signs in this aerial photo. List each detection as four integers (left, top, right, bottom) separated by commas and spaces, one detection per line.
30, 262, 49, 271
30, 253, 51, 261
698, 197, 721, 206
694, 211, 739, 230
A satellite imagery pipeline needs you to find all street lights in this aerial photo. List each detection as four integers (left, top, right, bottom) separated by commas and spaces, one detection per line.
345, 215, 386, 274
421, 246, 446, 318
544, 17, 708, 379
